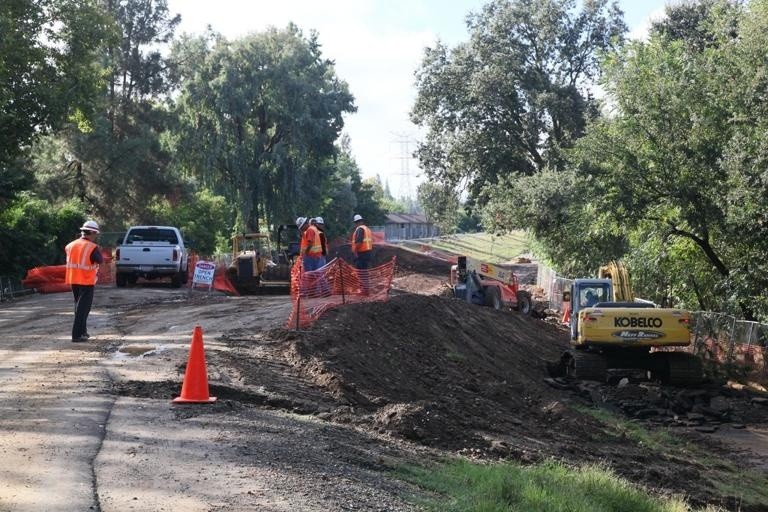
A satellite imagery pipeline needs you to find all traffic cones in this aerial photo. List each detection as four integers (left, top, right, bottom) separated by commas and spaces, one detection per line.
173, 325, 217, 404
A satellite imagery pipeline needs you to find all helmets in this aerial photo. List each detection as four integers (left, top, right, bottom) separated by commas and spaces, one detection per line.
295, 217, 324, 230
79, 220, 100, 234
353, 214, 363, 222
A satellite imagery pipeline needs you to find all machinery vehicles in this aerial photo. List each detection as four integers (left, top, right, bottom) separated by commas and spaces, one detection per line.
450, 256, 533, 315
559, 260, 704, 388
228, 224, 303, 296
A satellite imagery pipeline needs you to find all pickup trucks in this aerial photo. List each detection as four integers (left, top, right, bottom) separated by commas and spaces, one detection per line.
115, 225, 188, 288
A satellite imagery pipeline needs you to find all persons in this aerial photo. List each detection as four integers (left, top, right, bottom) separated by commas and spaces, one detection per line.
584, 289, 598, 306
295, 213, 372, 297
65, 220, 104, 342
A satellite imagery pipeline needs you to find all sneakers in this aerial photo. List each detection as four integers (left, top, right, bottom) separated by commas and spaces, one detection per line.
72, 335, 88, 342
